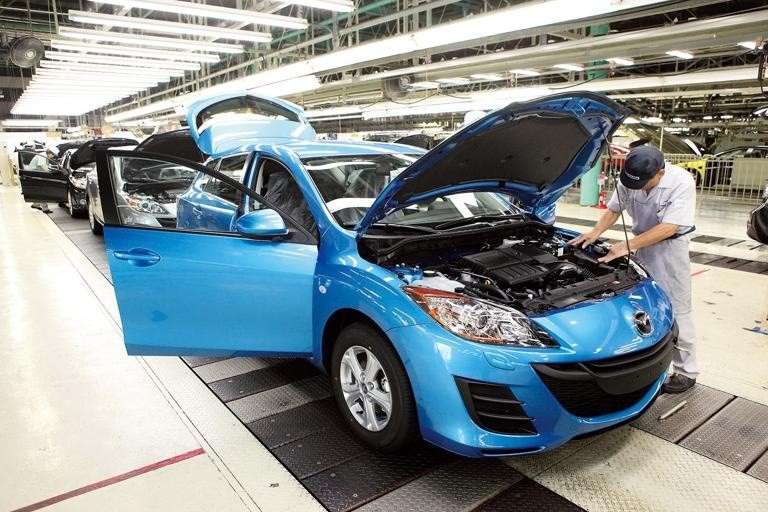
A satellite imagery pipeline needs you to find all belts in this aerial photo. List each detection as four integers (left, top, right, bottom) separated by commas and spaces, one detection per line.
661, 226, 695, 239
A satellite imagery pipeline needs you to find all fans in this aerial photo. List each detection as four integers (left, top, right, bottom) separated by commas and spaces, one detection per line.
2, 32, 45, 70
383, 60, 415, 99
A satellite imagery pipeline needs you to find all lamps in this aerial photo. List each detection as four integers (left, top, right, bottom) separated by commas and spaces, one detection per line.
417, 74, 499, 89
606, 58, 633, 67
666, 50, 693, 60
510, 69, 538, 76
555, 64, 583, 72
10, 0, 359, 117
739, 41, 762, 51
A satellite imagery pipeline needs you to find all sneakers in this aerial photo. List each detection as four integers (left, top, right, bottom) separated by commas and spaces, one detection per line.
663, 374, 696, 394
30, 205, 53, 213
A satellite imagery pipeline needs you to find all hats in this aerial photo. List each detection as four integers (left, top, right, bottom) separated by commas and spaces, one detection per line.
620, 145, 665, 189
49, 146, 60, 156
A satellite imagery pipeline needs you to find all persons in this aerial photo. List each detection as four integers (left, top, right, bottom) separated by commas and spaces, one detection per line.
25, 145, 59, 214
566, 143, 702, 395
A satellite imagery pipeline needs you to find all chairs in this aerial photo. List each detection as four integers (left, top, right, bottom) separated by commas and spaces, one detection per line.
338, 166, 387, 229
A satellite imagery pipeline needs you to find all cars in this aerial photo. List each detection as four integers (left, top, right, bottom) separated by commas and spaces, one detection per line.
672, 144, 768, 188
